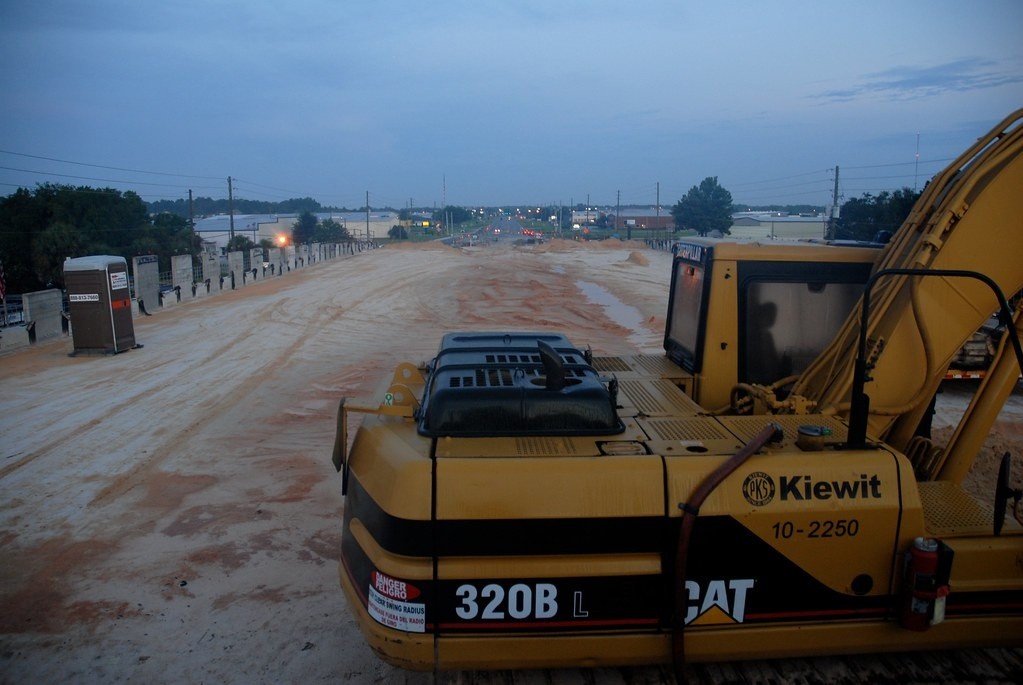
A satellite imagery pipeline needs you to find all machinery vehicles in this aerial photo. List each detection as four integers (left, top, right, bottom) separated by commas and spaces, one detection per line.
331, 106, 1023, 684
